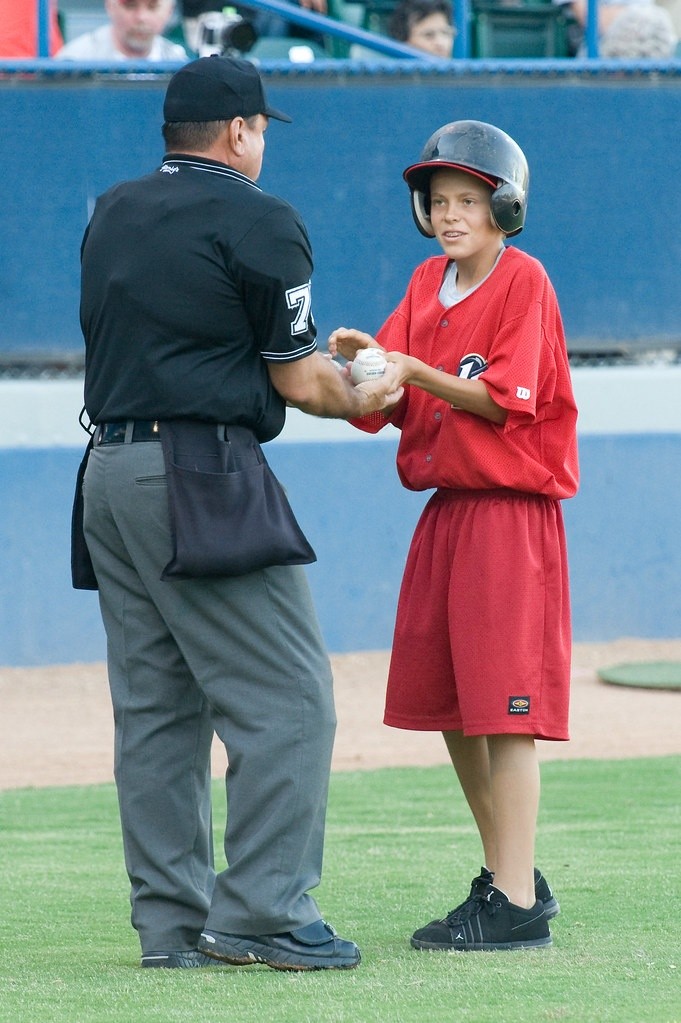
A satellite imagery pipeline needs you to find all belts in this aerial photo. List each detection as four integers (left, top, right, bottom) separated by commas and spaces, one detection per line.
99, 421, 162, 444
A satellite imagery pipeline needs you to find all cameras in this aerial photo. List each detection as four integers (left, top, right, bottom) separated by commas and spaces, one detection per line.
194, 10, 257, 58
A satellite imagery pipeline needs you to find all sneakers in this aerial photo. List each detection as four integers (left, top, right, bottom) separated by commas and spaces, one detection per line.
426, 866, 560, 926
411, 881, 554, 951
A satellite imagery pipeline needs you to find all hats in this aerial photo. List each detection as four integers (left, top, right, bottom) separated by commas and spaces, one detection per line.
163, 52, 293, 124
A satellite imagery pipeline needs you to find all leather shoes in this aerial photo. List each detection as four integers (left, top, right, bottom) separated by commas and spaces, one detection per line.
197, 917, 361, 971
140, 950, 226, 970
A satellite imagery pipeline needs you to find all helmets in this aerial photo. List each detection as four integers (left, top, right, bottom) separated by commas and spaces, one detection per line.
403, 119, 528, 239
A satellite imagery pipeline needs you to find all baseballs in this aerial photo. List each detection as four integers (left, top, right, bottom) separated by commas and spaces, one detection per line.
353, 348, 389, 386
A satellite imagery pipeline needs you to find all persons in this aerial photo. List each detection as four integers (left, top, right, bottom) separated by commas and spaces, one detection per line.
55, 0, 681, 970
